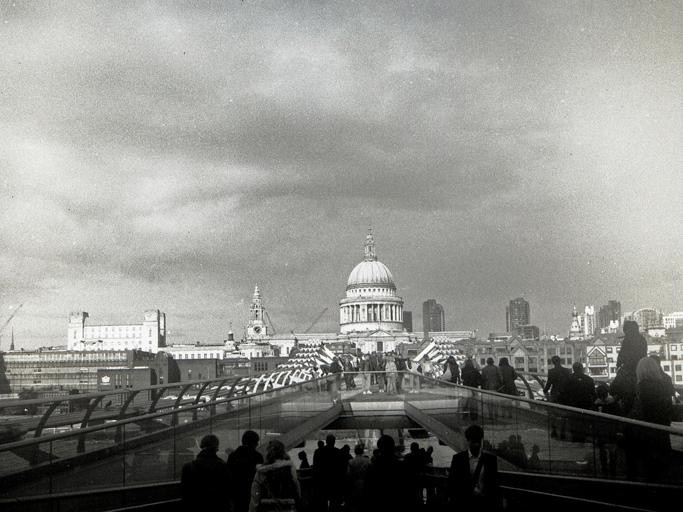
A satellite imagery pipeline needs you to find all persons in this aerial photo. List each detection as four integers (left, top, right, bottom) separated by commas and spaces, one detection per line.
484, 434, 543, 471
320, 351, 518, 422
542, 320, 675, 482
298, 434, 434, 511
179, 431, 301, 510
451, 427, 498, 510
